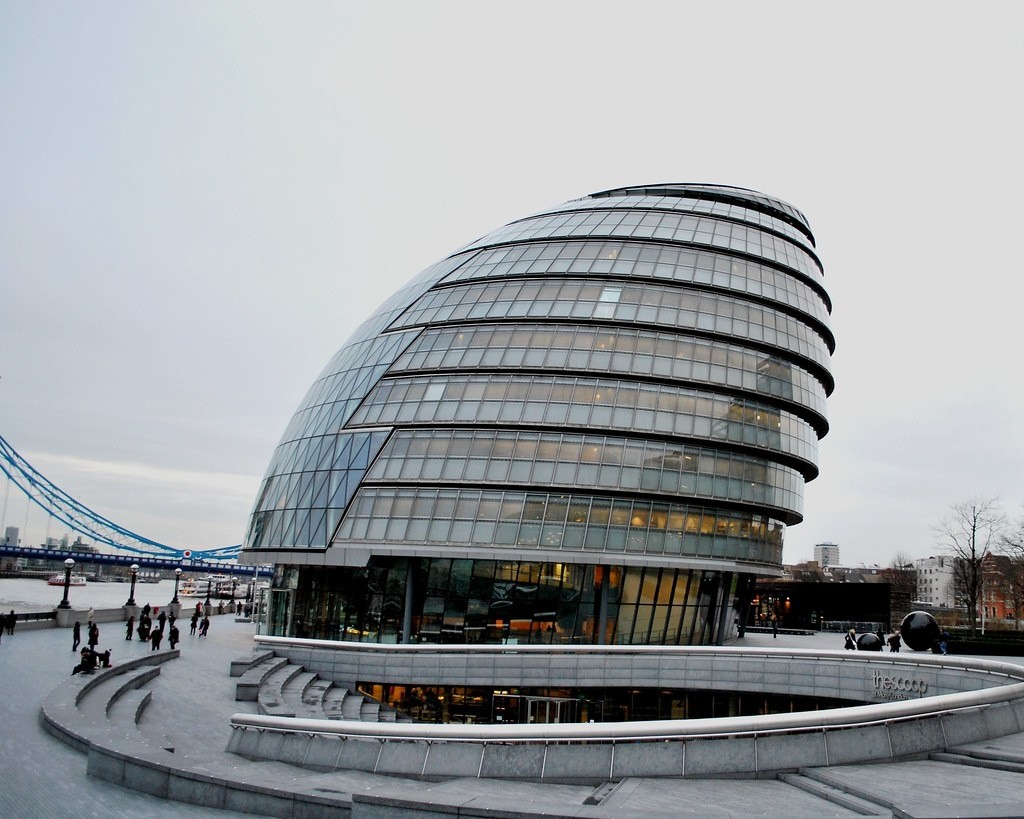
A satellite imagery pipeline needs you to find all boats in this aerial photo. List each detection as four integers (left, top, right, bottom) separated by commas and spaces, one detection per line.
179, 574, 266, 599
47, 573, 87, 586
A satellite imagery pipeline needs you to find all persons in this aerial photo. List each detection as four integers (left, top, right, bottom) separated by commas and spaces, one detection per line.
0, 610, 16, 637
73, 622, 81, 651
771, 617, 778, 638
877, 628, 884, 651
169, 626, 179, 649
73, 647, 112, 675
126, 616, 134, 640
219, 600, 226, 614
190, 602, 209, 639
149, 626, 162, 651
88, 607, 94, 628
157, 613, 166, 633
844, 629, 856, 650
236, 601, 258, 617
88, 624, 98, 650
169, 612, 175, 634
939, 629, 947, 655
137, 603, 152, 642
887, 629, 901, 653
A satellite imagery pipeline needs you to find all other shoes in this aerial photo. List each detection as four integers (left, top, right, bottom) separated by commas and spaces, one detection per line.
11, 633, 13, 635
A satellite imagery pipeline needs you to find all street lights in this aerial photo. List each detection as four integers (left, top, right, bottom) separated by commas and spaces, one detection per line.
248, 578, 256, 601
228, 576, 237, 604
171, 567, 182, 603
204, 575, 213, 605
127, 564, 139, 606
57, 558, 76, 608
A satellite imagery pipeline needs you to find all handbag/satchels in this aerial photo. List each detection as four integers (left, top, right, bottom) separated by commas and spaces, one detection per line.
94, 628, 98, 638
5, 624, 10, 629
